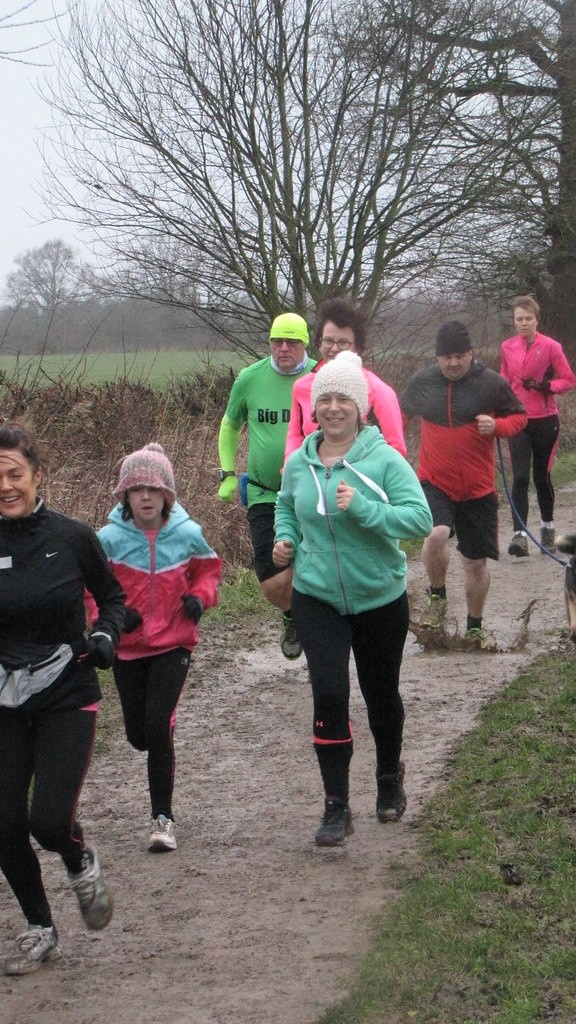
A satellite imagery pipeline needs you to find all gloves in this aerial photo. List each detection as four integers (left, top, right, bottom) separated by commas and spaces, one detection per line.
182, 594, 204, 626
87, 632, 115, 670
520, 375, 551, 393
123, 608, 142, 634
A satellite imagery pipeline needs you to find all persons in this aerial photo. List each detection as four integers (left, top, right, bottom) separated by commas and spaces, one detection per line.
269, 349, 434, 845
402, 321, 528, 643
500, 290, 576, 556
86, 442, 222, 849
279, 296, 407, 478
218, 311, 318, 661
0, 422, 126, 976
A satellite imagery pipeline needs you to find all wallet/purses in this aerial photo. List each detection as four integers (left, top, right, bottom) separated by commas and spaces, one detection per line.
240, 474, 248, 505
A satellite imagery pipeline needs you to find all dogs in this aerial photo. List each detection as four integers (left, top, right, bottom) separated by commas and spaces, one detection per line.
555, 533, 576, 644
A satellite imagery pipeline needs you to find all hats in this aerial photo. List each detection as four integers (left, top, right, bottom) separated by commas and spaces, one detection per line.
113, 443, 176, 509
311, 351, 368, 423
436, 321, 470, 356
270, 313, 309, 348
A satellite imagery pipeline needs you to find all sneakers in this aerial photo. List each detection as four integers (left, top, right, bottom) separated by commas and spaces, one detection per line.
375, 762, 407, 823
423, 594, 448, 628
4, 924, 58, 975
541, 527, 557, 555
66, 844, 113, 932
282, 615, 304, 660
509, 531, 530, 558
147, 814, 177, 852
315, 797, 355, 847
466, 628, 484, 643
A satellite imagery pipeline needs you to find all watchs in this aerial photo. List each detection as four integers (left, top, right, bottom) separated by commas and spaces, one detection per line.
217, 469, 235, 482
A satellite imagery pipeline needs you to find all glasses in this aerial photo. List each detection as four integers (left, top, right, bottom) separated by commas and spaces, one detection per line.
319, 336, 354, 351
271, 339, 301, 346
